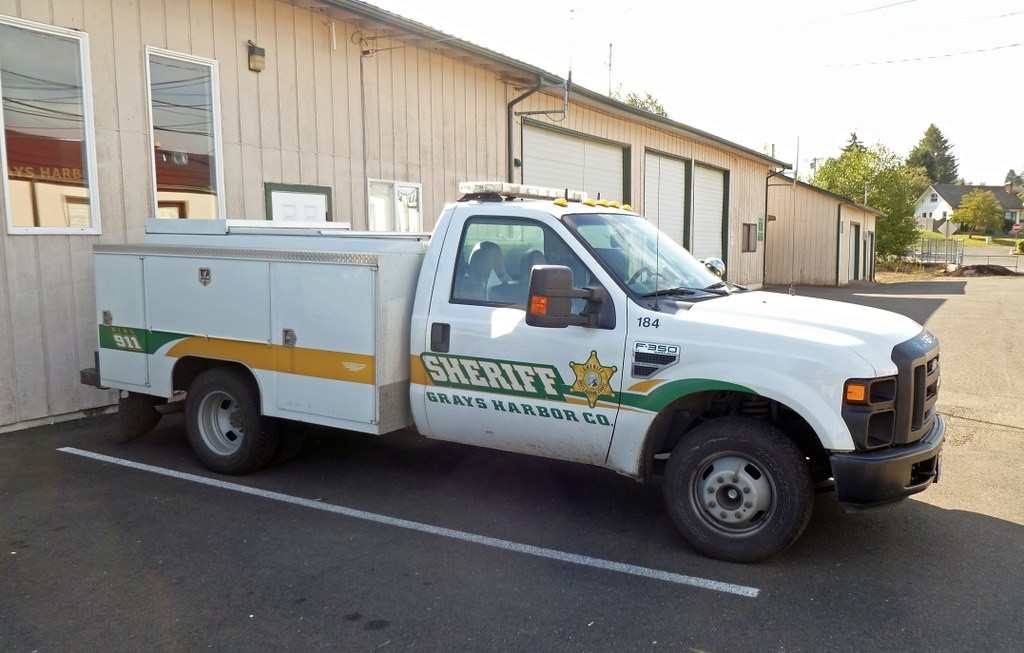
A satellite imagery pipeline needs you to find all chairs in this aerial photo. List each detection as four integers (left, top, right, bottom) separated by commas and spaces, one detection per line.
517, 249, 547, 306
462, 241, 504, 302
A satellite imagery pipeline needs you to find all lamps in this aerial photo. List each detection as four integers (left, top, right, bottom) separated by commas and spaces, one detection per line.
248, 38, 266, 72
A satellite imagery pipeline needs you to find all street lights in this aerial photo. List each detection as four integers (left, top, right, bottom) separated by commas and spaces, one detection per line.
864, 182, 870, 204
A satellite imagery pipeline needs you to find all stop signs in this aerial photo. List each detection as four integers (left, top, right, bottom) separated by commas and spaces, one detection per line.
1013, 224, 1022, 232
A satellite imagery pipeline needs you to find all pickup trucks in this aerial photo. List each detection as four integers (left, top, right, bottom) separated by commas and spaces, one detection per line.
88, 181, 948, 566
